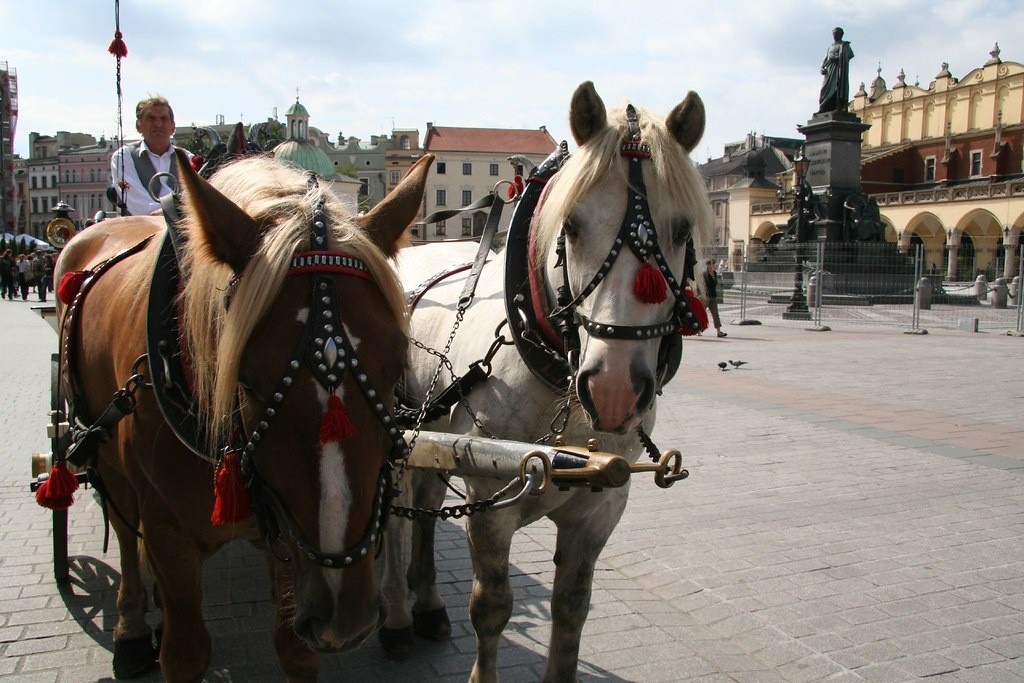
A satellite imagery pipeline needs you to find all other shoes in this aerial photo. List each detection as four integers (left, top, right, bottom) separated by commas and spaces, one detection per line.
717, 332, 727, 337
23, 296, 27, 300
8, 294, 12, 300
39, 299, 46, 302
2, 292, 5, 299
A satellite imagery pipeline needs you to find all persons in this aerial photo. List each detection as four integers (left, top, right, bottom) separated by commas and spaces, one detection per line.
843, 185, 887, 242
0, 249, 59, 302
930, 260, 946, 274
787, 180, 821, 235
688, 259, 731, 337
819, 27, 855, 113
111, 98, 195, 215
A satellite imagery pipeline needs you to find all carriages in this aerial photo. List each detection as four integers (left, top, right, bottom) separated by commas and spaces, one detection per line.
29, 78, 723, 683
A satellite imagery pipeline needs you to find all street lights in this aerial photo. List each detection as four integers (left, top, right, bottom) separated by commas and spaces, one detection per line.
786, 144, 809, 312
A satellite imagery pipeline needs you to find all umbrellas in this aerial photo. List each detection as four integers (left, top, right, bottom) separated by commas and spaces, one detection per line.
0, 232, 49, 250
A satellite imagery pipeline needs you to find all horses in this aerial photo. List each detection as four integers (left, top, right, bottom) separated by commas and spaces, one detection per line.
52, 80, 719, 683
